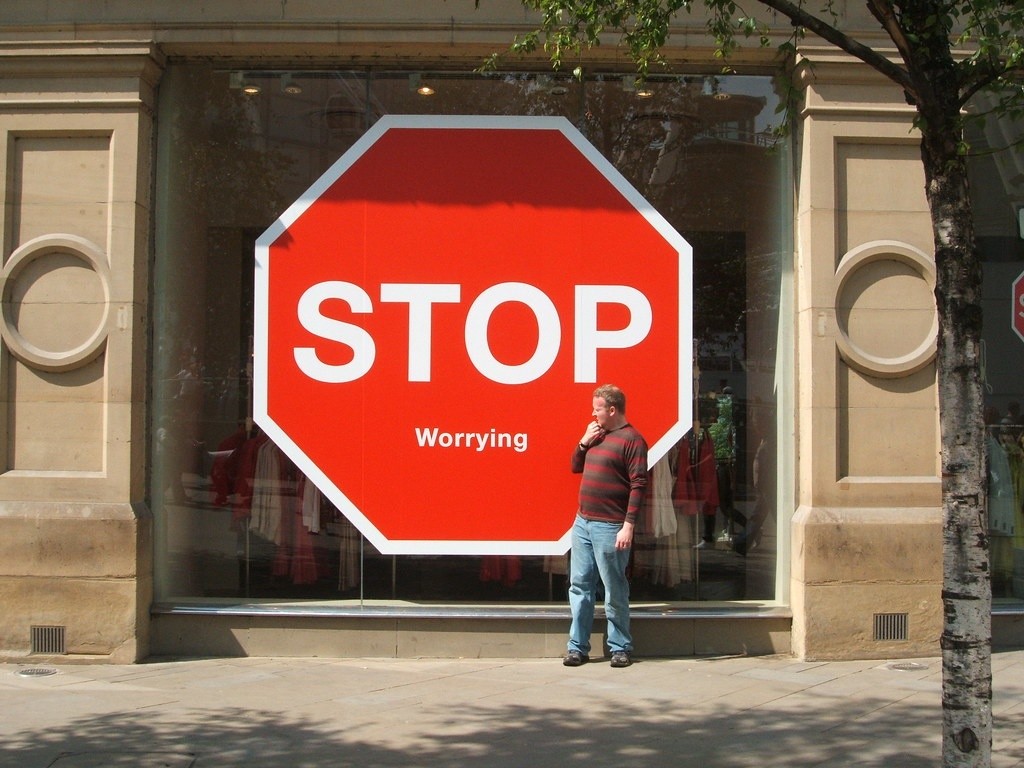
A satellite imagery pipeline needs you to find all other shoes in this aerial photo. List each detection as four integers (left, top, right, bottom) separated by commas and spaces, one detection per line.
718, 534, 732, 541
725, 541, 735, 551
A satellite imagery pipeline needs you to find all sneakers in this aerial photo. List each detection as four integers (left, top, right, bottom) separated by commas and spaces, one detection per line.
753, 526, 763, 548
692, 539, 715, 550
563, 650, 589, 666
611, 652, 632, 666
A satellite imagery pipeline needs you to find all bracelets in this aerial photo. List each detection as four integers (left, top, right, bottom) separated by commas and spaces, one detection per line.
578, 441, 589, 448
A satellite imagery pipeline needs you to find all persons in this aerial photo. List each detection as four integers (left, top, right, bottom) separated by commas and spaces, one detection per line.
689, 442, 765, 552
563, 384, 648, 667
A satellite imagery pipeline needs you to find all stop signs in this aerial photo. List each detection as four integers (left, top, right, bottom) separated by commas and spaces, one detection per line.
253, 117, 692, 556
